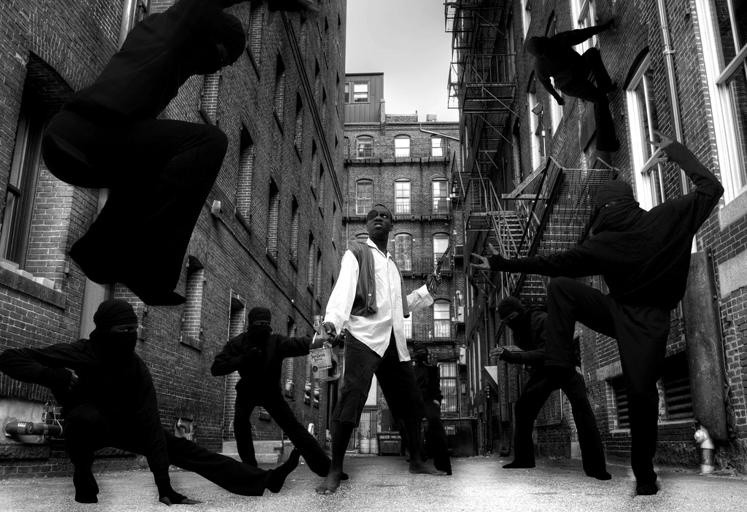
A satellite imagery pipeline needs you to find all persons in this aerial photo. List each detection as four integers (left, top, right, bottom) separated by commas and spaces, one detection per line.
526, 14, 625, 153
0, 298, 303, 507
467, 129, 726, 499
210, 304, 349, 479
39, 2, 316, 307
405, 342, 443, 466
487, 295, 611, 481
309, 202, 450, 494
